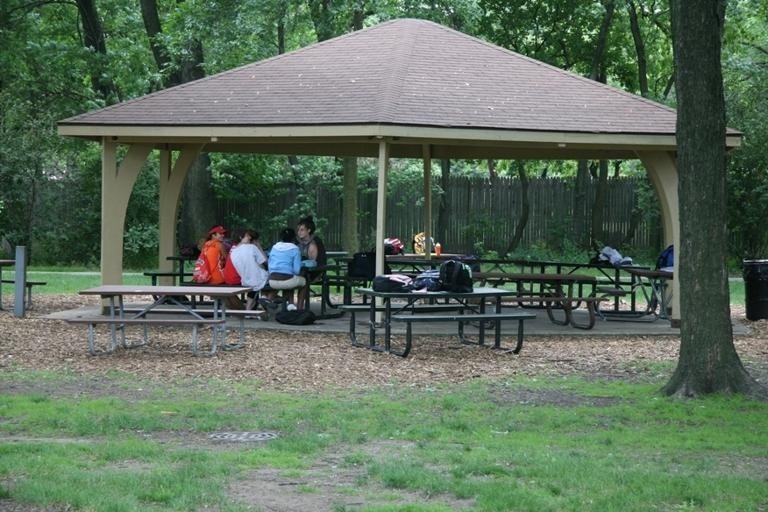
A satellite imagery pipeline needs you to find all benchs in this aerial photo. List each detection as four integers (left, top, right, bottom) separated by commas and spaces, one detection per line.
1, 279, 46, 310
65, 316, 225, 357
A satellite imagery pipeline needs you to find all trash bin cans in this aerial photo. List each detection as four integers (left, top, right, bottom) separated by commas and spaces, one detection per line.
743, 261, 768, 321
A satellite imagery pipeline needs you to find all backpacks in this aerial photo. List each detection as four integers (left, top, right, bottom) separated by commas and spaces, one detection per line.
348, 232, 473, 293
276, 310, 315, 325
193, 239, 222, 283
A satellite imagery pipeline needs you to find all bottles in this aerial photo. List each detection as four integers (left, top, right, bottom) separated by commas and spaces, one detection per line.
435, 241, 441, 258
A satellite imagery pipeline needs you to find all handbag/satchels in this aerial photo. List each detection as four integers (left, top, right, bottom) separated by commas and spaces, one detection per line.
222, 253, 241, 285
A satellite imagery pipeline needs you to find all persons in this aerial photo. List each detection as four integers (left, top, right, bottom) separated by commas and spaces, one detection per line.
201, 215, 327, 319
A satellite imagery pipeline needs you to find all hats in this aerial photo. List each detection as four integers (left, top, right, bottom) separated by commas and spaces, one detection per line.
208, 225, 228, 237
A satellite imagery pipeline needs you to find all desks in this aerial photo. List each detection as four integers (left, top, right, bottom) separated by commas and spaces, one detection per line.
79, 254, 673, 358
0, 260, 16, 310
